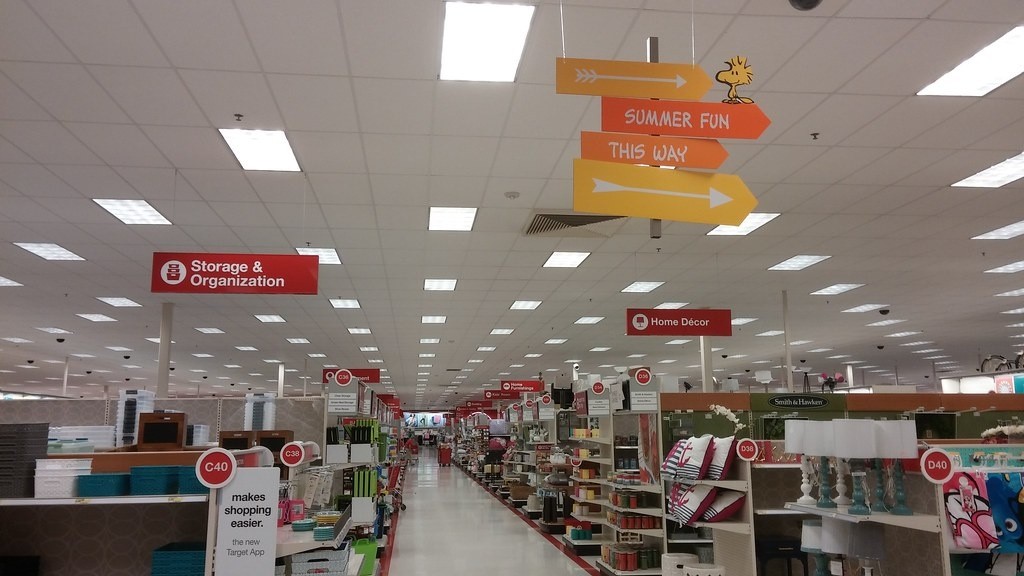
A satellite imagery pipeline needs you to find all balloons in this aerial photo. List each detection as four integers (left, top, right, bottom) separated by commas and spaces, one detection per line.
817, 373, 827, 383
835, 372, 844, 382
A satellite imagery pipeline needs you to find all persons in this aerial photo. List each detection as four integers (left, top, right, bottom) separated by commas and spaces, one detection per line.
453, 426, 463, 446
427, 429, 431, 435
406, 414, 417, 427
420, 415, 428, 426
405, 437, 416, 454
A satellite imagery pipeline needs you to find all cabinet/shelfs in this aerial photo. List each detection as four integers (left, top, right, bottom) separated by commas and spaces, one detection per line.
327, 411, 400, 520
751, 463, 814, 514
455, 394, 1024, 576
661, 459, 757, 576
275, 504, 364, 576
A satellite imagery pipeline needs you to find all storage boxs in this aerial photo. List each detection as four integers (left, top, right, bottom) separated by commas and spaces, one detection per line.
0, 389, 210, 576
326, 378, 396, 425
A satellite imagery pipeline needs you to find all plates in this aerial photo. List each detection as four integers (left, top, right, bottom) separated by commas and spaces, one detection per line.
317, 515, 340, 526
314, 526, 334, 541
292, 519, 317, 531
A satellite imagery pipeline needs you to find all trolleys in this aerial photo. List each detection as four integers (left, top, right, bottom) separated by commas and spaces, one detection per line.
383, 488, 406, 512
438, 444, 452, 466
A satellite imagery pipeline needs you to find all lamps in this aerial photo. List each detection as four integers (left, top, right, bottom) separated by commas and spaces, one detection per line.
889, 420, 919, 515
805, 421, 836, 506
850, 521, 884, 576
785, 420, 816, 503
876, 420, 903, 514
821, 515, 850, 576
832, 419, 876, 515
800, 519, 830, 576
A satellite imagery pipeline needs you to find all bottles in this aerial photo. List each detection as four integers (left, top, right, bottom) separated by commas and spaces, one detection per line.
573, 467, 595, 479
615, 456, 638, 469
609, 490, 651, 509
573, 503, 590, 516
571, 428, 600, 438
607, 511, 662, 529
601, 544, 661, 571
803, 372, 811, 393
606, 470, 640, 485
575, 486, 595, 500
573, 448, 590, 459
614, 435, 638, 447
570, 529, 592, 541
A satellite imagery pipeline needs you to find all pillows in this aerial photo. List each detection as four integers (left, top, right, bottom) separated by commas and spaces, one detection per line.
707, 434, 737, 480
668, 482, 691, 517
677, 435, 714, 478
703, 489, 746, 522
673, 484, 721, 529
660, 439, 688, 478
